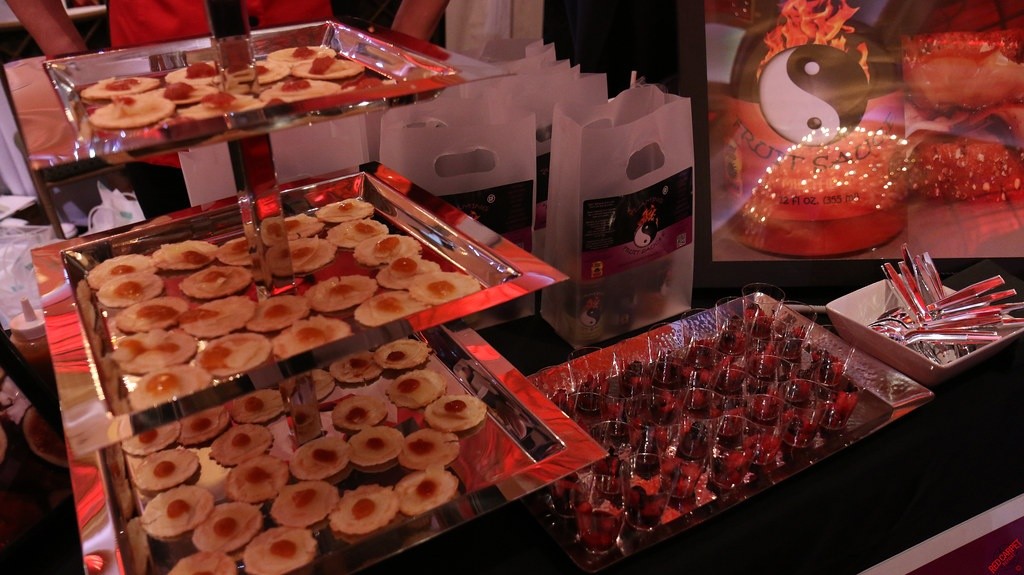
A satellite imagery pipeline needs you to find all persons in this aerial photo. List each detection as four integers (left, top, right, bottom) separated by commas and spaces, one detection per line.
5, 0, 454, 222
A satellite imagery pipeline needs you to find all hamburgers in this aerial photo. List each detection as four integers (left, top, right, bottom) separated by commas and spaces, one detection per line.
726, 121, 914, 257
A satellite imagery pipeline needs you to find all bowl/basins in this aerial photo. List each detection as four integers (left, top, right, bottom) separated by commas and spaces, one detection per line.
825, 273, 1024, 388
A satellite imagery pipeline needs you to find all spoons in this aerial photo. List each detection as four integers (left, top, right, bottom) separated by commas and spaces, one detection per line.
867, 241, 1024, 366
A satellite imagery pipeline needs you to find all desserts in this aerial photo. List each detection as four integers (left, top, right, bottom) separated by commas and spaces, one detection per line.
80, 45, 858, 575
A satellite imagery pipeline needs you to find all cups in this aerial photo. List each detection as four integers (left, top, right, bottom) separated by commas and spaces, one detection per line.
523, 282, 866, 565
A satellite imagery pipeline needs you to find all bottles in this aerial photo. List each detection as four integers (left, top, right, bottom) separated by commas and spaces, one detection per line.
9, 298, 51, 367
